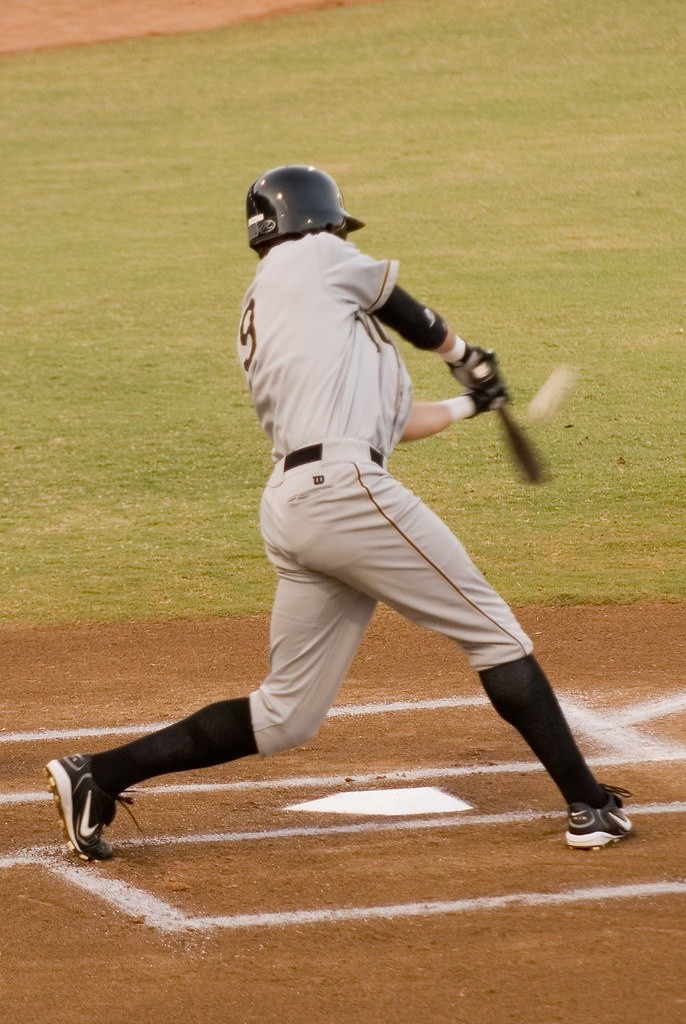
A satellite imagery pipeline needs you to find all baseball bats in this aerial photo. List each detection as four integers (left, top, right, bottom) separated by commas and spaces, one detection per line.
471, 358, 554, 488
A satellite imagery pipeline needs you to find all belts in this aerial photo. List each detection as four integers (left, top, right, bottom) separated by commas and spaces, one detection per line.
284, 443, 386, 475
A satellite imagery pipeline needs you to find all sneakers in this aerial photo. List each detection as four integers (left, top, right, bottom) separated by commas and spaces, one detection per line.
568, 783, 632, 849
43, 754, 143, 862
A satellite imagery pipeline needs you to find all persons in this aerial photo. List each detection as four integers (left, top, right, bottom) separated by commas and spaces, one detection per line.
42, 167, 632, 865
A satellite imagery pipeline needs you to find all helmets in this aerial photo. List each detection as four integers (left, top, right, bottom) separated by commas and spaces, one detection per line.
247, 166, 365, 246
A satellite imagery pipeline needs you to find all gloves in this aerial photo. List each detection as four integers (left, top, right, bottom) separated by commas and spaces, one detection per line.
448, 339, 499, 389
463, 391, 506, 417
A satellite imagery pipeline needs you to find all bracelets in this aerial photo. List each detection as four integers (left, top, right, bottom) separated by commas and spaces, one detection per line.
437, 336, 466, 365
440, 395, 477, 423
